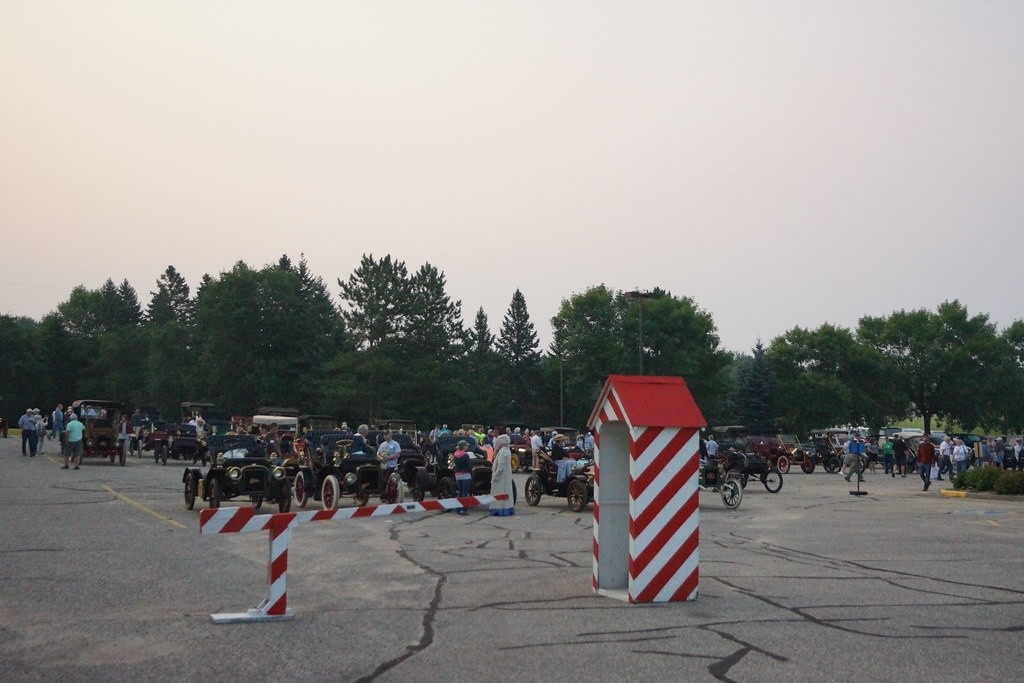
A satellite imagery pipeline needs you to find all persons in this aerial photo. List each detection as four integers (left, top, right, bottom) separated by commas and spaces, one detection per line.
45, 403, 73, 442
429, 422, 453, 464
130, 408, 142, 434
185, 408, 205, 437
916, 432, 1024, 492
835, 433, 908, 482
118, 415, 133, 455
354, 424, 369, 444
235, 423, 294, 460
341, 421, 353, 434
455, 426, 501, 451
18, 407, 49, 458
490, 434, 516, 516
61, 413, 85, 470
505, 426, 547, 469
80, 404, 107, 419
547, 430, 594, 485
453, 439, 472, 516
376, 430, 402, 497
699, 434, 744, 492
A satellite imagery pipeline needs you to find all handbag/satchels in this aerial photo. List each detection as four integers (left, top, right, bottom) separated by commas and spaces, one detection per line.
38, 423, 47, 437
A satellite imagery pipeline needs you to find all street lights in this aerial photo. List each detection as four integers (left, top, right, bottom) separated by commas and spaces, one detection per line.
624, 291, 658, 376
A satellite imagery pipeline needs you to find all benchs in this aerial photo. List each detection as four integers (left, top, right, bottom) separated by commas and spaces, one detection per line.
716, 444, 745, 458
165, 423, 487, 462
79, 418, 112, 427
747, 435, 770, 449
538, 448, 580, 471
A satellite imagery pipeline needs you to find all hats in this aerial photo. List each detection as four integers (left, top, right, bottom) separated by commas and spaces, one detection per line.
457, 440, 469, 446
552, 431, 557, 434
553, 434, 567, 441
32, 408, 40, 412
26, 409, 34, 413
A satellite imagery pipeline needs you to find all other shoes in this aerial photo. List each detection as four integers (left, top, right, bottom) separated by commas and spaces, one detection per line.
937, 478, 944, 481
23, 451, 27, 456
30, 452, 35, 457
902, 475, 906, 477
859, 480, 864, 482
844, 477, 850, 482
892, 472, 895, 477
923, 488, 928, 491
928, 482, 932, 486
457, 509, 469, 514
62, 465, 69, 469
47, 435, 51, 440
839, 472, 844, 477
74, 466, 79, 470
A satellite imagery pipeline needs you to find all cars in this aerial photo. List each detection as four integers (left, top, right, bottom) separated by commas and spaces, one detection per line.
129, 401, 595, 513
59, 400, 126, 466
699, 425, 1024, 510
